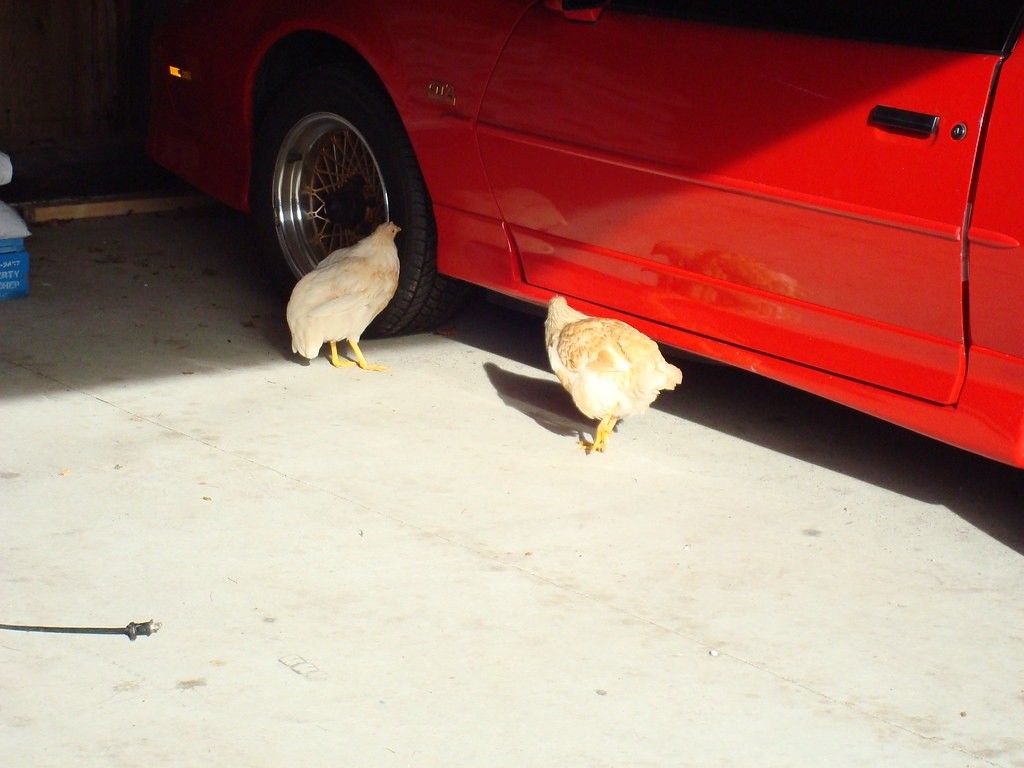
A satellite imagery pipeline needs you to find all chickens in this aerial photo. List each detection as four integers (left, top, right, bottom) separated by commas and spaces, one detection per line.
286, 222, 401, 372
545, 295, 682, 453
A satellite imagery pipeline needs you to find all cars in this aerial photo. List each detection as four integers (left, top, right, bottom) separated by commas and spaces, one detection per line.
140, 0, 1024, 469
